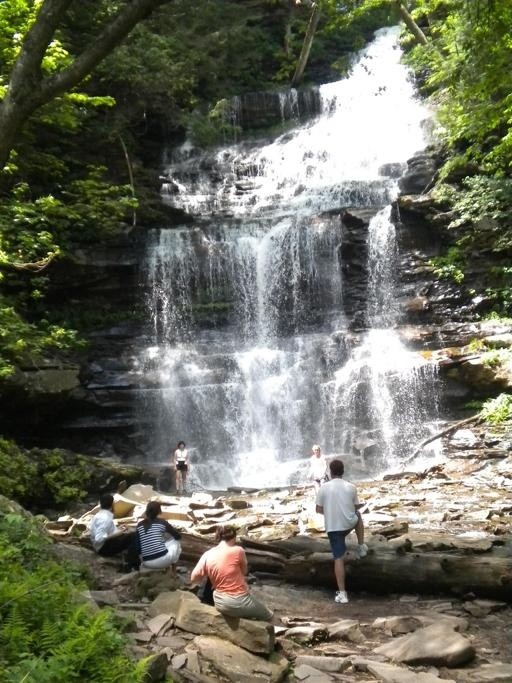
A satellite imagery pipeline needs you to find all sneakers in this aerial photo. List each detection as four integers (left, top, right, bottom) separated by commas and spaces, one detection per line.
357, 544, 369, 558
333, 589, 349, 604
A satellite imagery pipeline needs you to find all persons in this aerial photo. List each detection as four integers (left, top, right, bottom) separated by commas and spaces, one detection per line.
89, 494, 142, 570
308, 444, 328, 494
173, 440, 190, 494
190, 522, 283, 626
315, 458, 369, 603
135, 499, 188, 573
